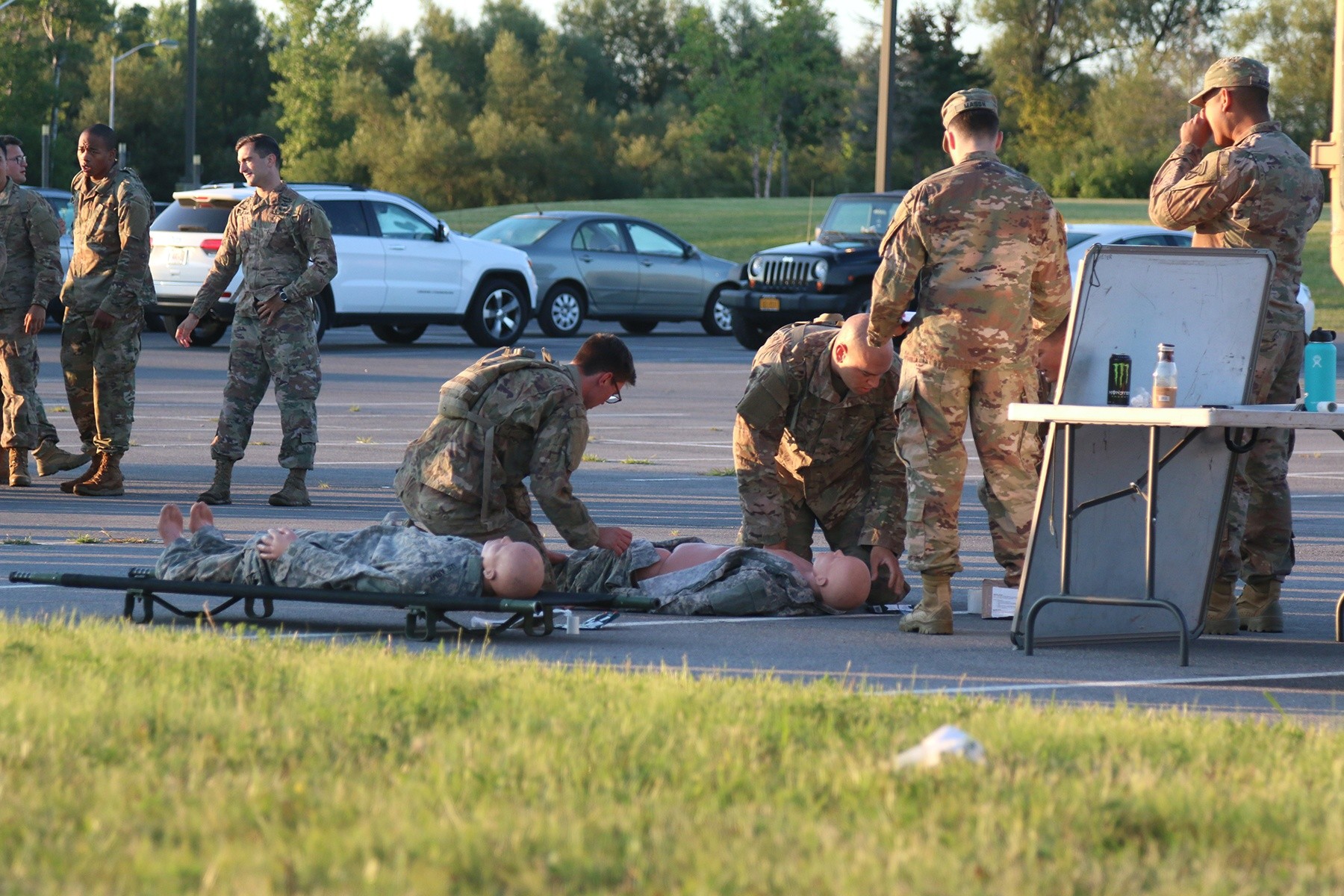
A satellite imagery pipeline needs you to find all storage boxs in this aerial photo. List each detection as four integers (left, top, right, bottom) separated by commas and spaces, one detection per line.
979, 578, 1018, 618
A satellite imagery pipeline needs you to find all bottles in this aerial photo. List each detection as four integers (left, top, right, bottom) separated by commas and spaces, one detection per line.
1152, 342, 1178, 407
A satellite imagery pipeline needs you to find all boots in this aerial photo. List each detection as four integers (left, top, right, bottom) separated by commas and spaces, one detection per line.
897, 575, 954, 636
60, 450, 126, 496
1236, 576, 1285, 634
1203, 580, 1239, 636
8, 447, 31, 487
32, 439, 91, 477
197, 459, 233, 505
268, 468, 312, 507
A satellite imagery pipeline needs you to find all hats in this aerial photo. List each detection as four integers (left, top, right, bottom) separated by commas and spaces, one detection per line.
1189, 57, 1269, 109
941, 87, 998, 155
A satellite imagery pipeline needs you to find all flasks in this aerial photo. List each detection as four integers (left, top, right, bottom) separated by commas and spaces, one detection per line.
1303, 327, 1338, 412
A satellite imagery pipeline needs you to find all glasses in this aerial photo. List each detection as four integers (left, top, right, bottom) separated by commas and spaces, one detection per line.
7, 155, 27, 166
602, 366, 622, 405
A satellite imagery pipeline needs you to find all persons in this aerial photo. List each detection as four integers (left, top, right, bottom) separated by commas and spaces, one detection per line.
865, 87, 1073, 636
394, 333, 637, 598
1149, 58, 1327, 636
0, 136, 94, 487
1035, 314, 1067, 444
155, 496, 545, 599
61, 122, 155, 496
175, 136, 339, 505
549, 536, 868, 615
732, 314, 910, 610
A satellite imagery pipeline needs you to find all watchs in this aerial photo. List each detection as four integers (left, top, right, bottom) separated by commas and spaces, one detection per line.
279, 290, 289, 304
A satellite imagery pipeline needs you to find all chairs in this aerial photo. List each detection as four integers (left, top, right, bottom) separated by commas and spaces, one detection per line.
592, 226, 622, 250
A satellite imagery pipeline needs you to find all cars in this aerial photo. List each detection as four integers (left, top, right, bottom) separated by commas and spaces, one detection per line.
467, 211, 748, 336
1057, 223, 1315, 348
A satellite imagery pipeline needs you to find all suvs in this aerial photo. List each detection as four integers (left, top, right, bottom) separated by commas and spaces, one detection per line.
731, 188, 910, 352
10, 183, 540, 352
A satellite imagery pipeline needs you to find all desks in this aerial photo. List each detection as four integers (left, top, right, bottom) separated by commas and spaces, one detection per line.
1004, 402, 1343, 666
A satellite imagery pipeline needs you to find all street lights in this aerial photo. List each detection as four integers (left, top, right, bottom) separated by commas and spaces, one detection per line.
107, 37, 177, 129
